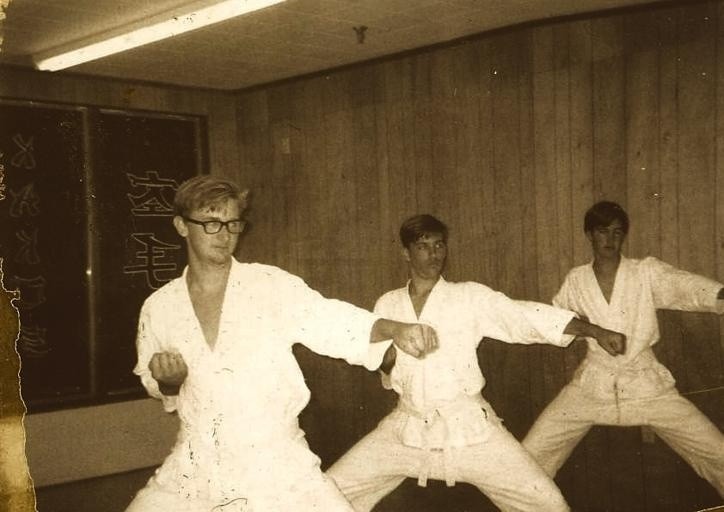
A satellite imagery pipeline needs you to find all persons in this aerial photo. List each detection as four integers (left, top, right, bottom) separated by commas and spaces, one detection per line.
127, 176, 438, 512
322, 213, 626, 511
521, 199, 724, 502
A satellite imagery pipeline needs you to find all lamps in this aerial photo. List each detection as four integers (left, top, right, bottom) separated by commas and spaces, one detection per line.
25, 1, 284, 76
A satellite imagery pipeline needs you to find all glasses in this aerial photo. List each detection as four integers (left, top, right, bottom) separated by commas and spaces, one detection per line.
181, 216, 248, 234
